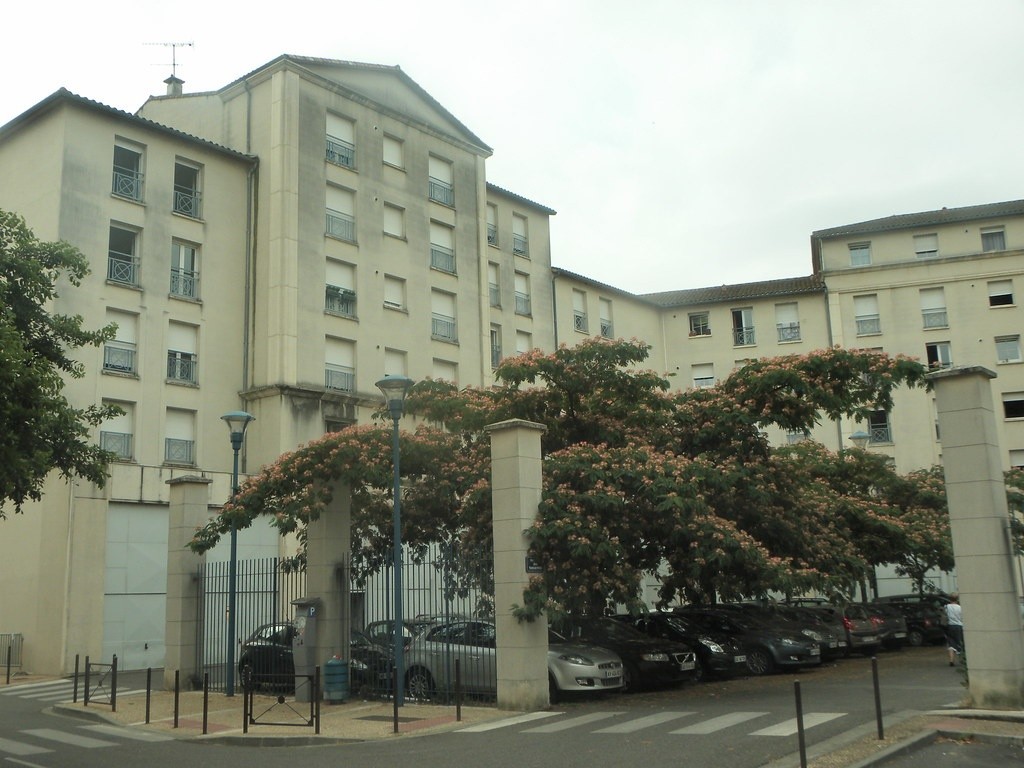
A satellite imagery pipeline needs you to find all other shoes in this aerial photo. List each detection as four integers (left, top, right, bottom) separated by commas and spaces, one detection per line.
950, 660, 954, 666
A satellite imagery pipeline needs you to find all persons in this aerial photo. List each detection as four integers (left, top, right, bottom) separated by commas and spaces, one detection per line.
942, 592, 964, 666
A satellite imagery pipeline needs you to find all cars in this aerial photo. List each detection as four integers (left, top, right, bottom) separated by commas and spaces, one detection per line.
548, 614, 699, 694
404, 614, 625, 705
364, 618, 439, 651
239, 620, 395, 693
631, 592, 955, 678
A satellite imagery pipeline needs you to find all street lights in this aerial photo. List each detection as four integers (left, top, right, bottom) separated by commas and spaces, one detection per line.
218, 409, 257, 698
846, 430, 878, 599
374, 375, 417, 708
